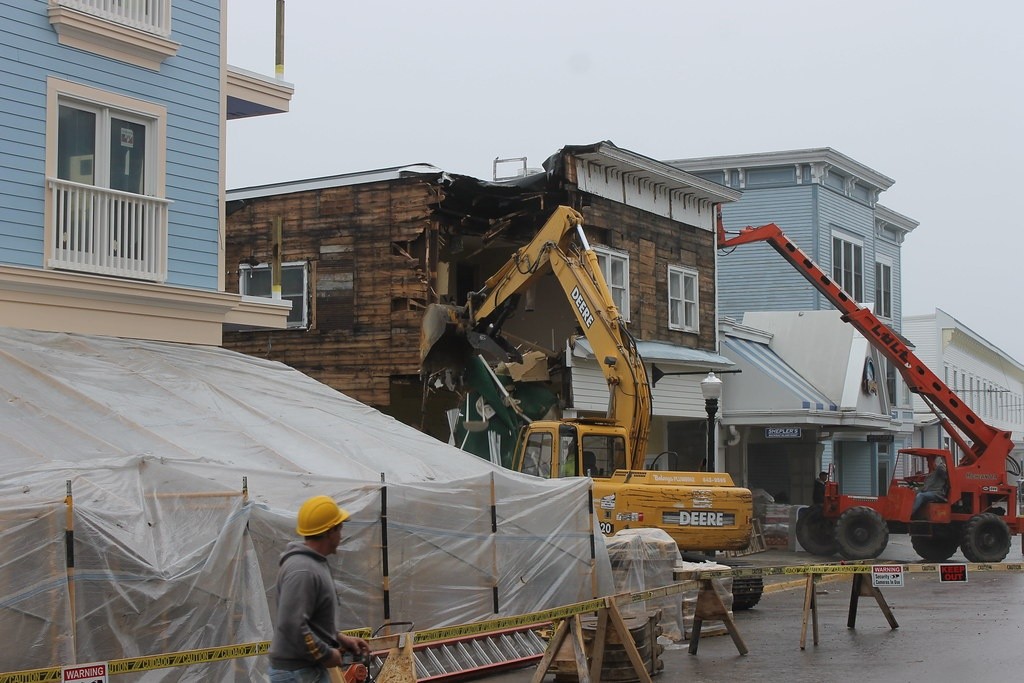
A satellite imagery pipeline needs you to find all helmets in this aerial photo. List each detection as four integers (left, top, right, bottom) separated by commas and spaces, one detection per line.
294, 495, 350, 537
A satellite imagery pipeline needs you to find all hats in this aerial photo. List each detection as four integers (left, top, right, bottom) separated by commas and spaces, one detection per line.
819, 472, 829, 477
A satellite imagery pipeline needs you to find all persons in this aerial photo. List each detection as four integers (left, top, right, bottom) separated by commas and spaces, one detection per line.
268, 496, 372, 683
541, 442, 576, 478
912, 455, 948, 513
813, 472, 829, 505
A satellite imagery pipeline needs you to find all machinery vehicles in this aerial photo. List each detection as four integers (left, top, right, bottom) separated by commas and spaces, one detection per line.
419, 186, 766, 616
715, 202, 1024, 565
